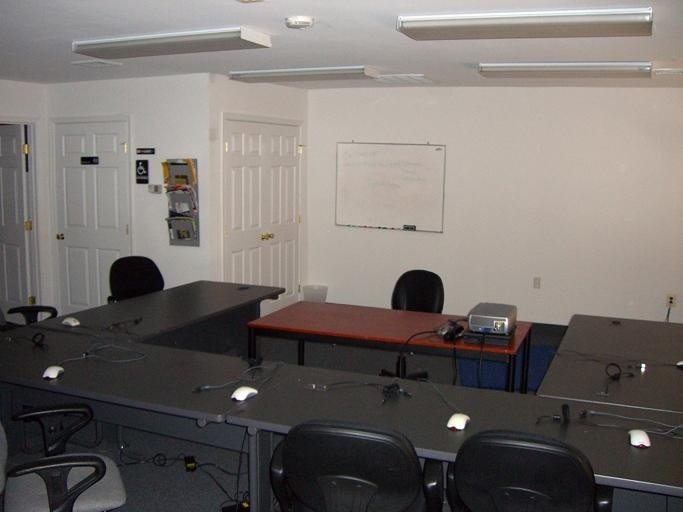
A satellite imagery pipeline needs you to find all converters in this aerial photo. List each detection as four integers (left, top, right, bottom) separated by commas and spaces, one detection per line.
185, 455, 197, 472
223, 501, 251, 512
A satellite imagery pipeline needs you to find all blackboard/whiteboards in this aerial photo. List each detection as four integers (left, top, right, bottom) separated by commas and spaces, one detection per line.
335, 141, 447, 233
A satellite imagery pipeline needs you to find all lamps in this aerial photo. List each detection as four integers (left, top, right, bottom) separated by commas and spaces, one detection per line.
478, 62, 654, 81
396, 7, 656, 41
227, 65, 380, 84
70, 27, 272, 62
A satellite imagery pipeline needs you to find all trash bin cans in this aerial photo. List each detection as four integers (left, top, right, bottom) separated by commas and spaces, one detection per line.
303, 284, 328, 303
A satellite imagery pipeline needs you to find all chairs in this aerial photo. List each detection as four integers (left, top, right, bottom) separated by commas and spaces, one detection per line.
0, 306, 54, 332
266, 418, 446, 510
445, 429, 612, 510
0, 404, 126, 512
105, 255, 165, 305
376, 268, 445, 380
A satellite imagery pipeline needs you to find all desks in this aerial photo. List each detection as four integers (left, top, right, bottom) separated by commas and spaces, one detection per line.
29, 280, 284, 357
0, 326, 302, 511
246, 301, 534, 392
534, 313, 683, 414
227, 365, 683, 511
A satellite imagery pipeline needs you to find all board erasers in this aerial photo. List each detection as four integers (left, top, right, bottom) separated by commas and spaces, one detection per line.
403, 225, 416, 231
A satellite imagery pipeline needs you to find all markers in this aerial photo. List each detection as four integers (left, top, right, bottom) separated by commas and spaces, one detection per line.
347, 225, 395, 229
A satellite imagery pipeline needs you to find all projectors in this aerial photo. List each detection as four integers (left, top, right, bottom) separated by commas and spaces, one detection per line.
468, 302, 517, 334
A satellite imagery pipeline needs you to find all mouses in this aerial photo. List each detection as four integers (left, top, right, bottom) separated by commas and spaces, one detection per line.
231, 385, 257, 401
42, 365, 64, 379
628, 429, 652, 449
446, 413, 470, 431
62, 318, 80, 327
